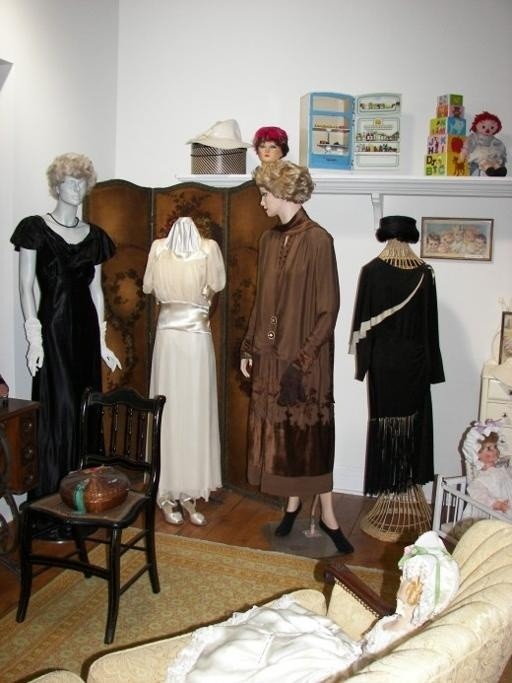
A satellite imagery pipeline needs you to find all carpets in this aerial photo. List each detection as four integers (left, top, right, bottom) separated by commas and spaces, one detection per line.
0, 526, 406, 682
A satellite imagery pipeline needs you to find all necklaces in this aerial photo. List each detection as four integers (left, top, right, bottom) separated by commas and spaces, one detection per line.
46, 212, 80, 227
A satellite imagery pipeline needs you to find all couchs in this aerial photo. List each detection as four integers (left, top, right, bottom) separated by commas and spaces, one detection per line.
32, 511, 511, 683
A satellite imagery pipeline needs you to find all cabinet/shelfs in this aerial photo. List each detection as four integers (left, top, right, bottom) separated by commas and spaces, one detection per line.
0, 395, 45, 556
476, 361, 512, 461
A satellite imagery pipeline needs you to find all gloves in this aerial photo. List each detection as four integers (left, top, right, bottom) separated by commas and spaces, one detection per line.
99, 319, 123, 373
23, 316, 46, 379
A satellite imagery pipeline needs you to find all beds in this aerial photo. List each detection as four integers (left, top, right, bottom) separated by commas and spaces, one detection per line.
430, 470, 512, 552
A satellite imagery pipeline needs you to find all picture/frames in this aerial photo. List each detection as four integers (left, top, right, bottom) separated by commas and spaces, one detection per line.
498, 310, 512, 365
420, 217, 495, 262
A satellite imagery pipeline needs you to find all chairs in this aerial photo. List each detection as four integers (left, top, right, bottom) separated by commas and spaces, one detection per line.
15, 384, 166, 648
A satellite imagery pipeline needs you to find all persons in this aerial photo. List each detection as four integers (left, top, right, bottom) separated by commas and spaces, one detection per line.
252, 126, 290, 163
348, 216, 449, 541
241, 159, 353, 555
144, 213, 229, 527
460, 417, 511, 522
457, 110, 506, 176
10, 153, 122, 500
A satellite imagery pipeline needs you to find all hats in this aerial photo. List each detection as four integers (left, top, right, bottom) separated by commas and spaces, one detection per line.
377, 213, 422, 245
185, 118, 255, 151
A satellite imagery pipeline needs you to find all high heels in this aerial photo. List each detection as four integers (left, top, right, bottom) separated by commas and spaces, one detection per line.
158, 493, 185, 526
178, 492, 208, 527
